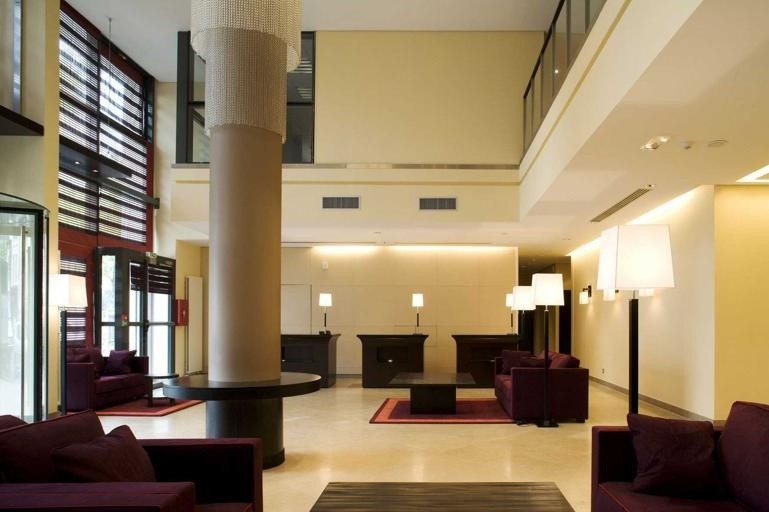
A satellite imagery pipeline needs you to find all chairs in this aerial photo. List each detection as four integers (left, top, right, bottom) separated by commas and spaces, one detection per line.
1, 415, 265, 512
591, 398, 769, 510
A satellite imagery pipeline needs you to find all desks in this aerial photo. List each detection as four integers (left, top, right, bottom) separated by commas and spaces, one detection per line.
451, 334, 521, 388
356, 335, 429, 388
281, 334, 341, 388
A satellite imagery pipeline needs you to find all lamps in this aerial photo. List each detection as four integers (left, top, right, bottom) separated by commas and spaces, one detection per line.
511, 287, 537, 350
319, 292, 333, 334
615, 223, 674, 415
412, 293, 424, 334
505, 293, 516, 334
531, 272, 564, 427
579, 285, 592, 304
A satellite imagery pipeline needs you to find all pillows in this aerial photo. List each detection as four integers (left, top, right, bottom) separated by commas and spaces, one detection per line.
627, 408, 736, 499
0, 410, 105, 482
44, 426, 157, 483
502, 350, 531, 374
519, 356, 552, 368
109, 349, 136, 374
67, 347, 113, 378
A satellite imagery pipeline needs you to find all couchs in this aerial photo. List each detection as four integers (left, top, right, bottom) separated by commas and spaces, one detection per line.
494, 350, 589, 423
65, 342, 152, 410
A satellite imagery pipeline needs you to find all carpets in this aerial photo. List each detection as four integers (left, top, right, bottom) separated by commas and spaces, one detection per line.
309, 480, 574, 512
67, 396, 206, 416
369, 397, 516, 423
